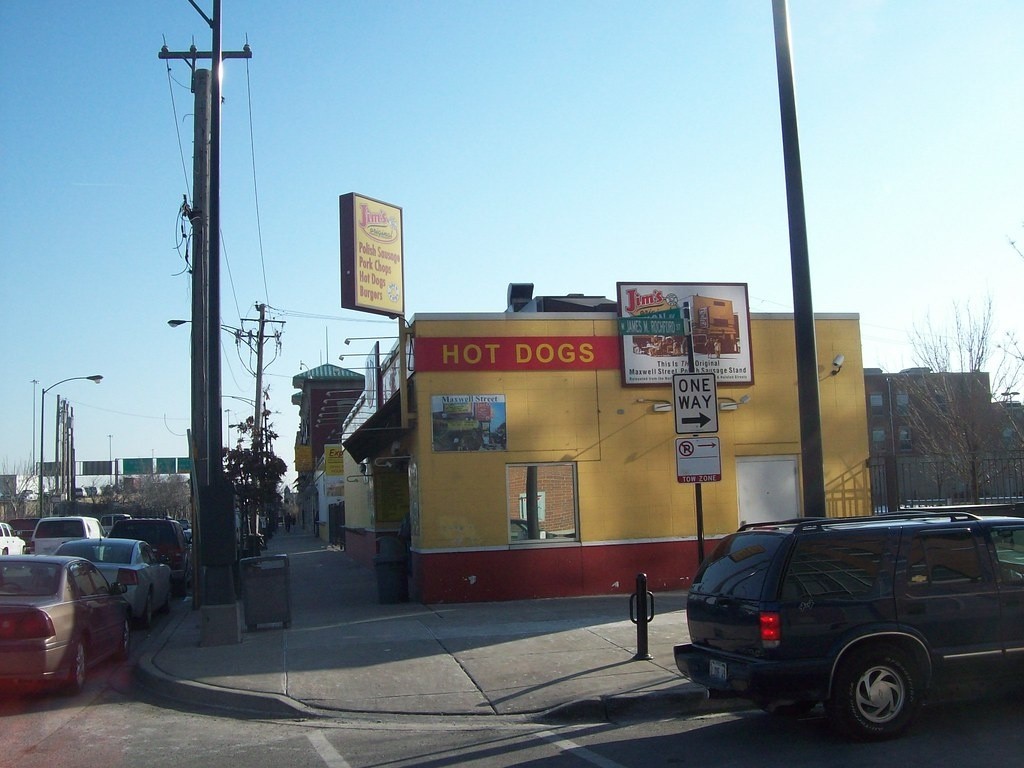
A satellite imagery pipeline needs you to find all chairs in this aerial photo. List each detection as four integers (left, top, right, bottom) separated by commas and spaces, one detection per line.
934, 550, 972, 581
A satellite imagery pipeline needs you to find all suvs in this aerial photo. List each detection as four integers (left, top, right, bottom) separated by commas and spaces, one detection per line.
103, 516, 188, 583
673, 505, 1023, 739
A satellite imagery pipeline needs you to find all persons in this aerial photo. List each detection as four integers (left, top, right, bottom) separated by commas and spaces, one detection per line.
315, 510, 320, 538
704, 338, 722, 359
284, 513, 297, 533
632, 336, 687, 357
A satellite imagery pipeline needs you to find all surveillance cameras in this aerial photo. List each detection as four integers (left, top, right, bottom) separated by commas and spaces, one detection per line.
832, 354, 845, 367
393, 441, 400, 452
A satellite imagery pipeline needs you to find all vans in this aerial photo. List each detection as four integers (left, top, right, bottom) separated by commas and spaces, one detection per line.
31, 515, 109, 565
99, 514, 133, 537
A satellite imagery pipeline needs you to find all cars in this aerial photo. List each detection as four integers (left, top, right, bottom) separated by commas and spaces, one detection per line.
0, 522, 30, 557
178, 519, 193, 544
47, 538, 175, 629
0, 555, 130, 697
6, 516, 41, 553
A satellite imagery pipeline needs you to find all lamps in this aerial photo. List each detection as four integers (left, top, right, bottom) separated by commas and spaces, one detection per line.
637, 398, 672, 411
315, 423, 359, 428
325, 390, 376, 396
321, 405, 369, 411
339, 350, 396, 361
328, 436, 346, 440
334, 363, 387, 374
317, 418, 367, 423
331, 429, 352, 434
323, 398, 376, 405
720, 394, 751, 410
344, 337, 399, 345
319, 412, 367, 417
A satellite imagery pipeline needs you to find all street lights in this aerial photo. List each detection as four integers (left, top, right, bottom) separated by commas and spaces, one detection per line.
228, 423, 274, 474
167, 318, 265, 554
38, 375, 106, 516
